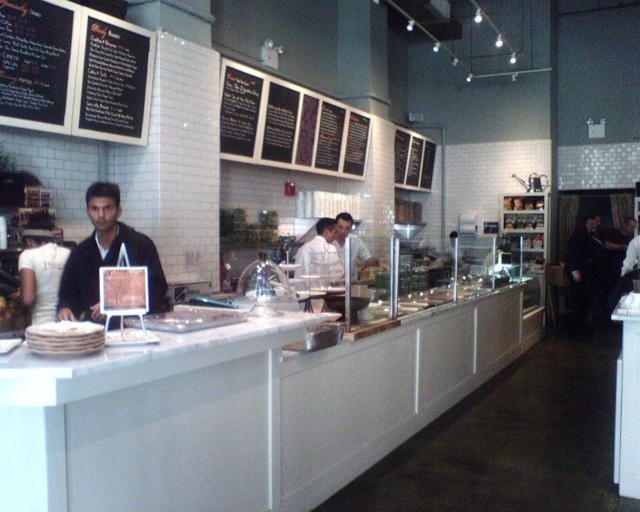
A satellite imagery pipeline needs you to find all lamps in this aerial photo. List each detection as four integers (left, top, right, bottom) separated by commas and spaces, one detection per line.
260, 37, 287, 70
371, 0, 477, 84
464, 0, 519, 65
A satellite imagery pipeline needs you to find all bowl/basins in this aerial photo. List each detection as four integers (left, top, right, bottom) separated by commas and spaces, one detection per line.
358, 301, 391, 321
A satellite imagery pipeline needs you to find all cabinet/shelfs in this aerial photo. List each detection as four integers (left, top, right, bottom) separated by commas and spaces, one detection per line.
498, 191, 550, 328
608, 293, 640, 502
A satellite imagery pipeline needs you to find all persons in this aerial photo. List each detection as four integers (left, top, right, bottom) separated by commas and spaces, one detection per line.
480, 237, 511, 277
336, 212, 379, 281
445, 231, 469, 275
561, 213, 640, 339
57, 181, 168, 331
6, 214, 70, 325
293, 217, 344, 294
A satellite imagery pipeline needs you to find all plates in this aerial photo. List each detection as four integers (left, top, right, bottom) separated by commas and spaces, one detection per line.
24, 319, 109, 356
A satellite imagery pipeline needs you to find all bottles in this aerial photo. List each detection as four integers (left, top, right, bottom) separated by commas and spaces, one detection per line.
532, 234, 542, 251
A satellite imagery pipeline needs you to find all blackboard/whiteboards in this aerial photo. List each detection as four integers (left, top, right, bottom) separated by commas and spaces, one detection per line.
394, 125, 437, 192
0, 0, 157, 147
220, 57, 372, 181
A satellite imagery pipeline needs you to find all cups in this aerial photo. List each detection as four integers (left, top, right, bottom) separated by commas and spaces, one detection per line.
310, 297, 325, 313
